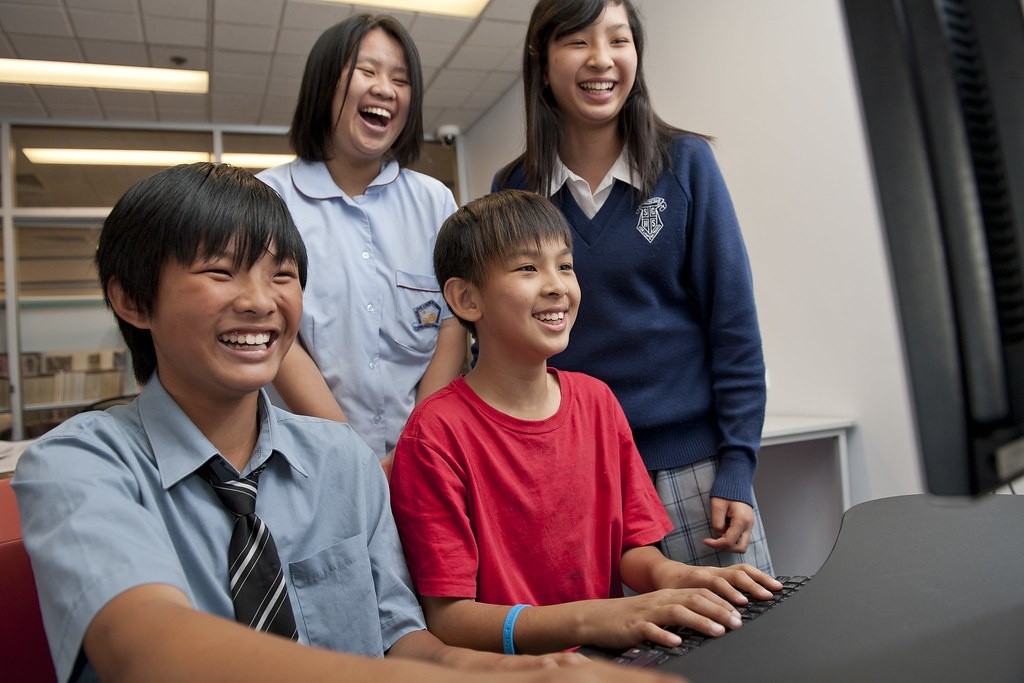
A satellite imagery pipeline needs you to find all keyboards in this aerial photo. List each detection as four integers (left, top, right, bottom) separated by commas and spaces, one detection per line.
568, 572, 822, 669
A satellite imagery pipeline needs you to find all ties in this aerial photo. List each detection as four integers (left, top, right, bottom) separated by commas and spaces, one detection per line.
192, 450, 302, 644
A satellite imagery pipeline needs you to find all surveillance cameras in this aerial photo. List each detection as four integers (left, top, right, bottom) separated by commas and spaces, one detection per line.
437, 125, 460, 147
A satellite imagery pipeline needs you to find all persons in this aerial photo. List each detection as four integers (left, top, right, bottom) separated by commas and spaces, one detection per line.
388, 189, 783, 654
10, 161, 688, 683
252, 15, 469, 475
490, 0, 774, 596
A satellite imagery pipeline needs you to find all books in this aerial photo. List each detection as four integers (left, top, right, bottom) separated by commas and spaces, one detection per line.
52, 369, 122, 422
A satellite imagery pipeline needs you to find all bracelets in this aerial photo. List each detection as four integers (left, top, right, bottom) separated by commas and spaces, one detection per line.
503, 605, 534, 656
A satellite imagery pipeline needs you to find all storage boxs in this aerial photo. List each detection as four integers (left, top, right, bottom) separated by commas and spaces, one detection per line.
39, 352, 80, 376
0, 351, 40, 380
80, 349, 126, 374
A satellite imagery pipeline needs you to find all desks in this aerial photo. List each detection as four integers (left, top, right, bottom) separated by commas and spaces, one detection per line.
760, 416, 859, 515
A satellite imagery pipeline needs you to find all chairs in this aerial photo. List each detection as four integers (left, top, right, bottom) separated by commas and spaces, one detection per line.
0, 476, 60, 683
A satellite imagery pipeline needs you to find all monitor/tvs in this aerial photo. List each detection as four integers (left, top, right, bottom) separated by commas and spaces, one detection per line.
837, 0, 1024, 497
